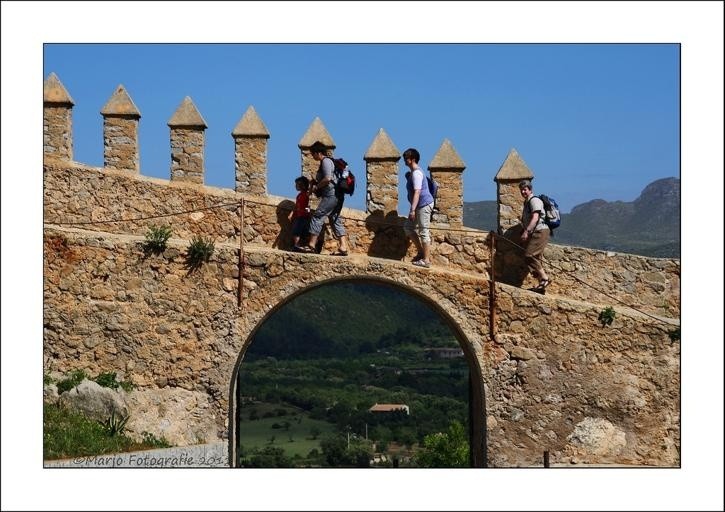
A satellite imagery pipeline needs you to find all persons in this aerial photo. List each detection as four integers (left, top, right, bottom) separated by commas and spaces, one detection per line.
294, 141, 347, 255
499, 179, 553, 293
287, 176, 315, 252
403, 148, 435, 269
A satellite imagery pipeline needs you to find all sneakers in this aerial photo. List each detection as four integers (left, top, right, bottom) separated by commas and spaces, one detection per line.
288, 245, 301, 252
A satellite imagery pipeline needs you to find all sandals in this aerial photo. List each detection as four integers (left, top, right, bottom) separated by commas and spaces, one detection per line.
526, 288, 546, 295
412, 259, 430, 268
296, 245, 318, 253
534, 277, 552, 293
329, 248, 348, 256
412, 253, 423, 262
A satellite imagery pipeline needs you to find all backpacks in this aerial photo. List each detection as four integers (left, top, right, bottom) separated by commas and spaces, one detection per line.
326, 157, 355, 196
527, 194, 560, 229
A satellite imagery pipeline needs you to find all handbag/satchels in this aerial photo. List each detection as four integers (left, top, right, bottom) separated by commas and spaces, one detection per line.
426, 177, 440, 211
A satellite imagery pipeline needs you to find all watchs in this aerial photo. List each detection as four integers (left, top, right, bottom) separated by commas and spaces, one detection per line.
526, 228, 534, 235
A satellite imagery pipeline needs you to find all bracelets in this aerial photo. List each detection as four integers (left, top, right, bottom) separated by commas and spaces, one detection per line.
411, 209, 416, 211
